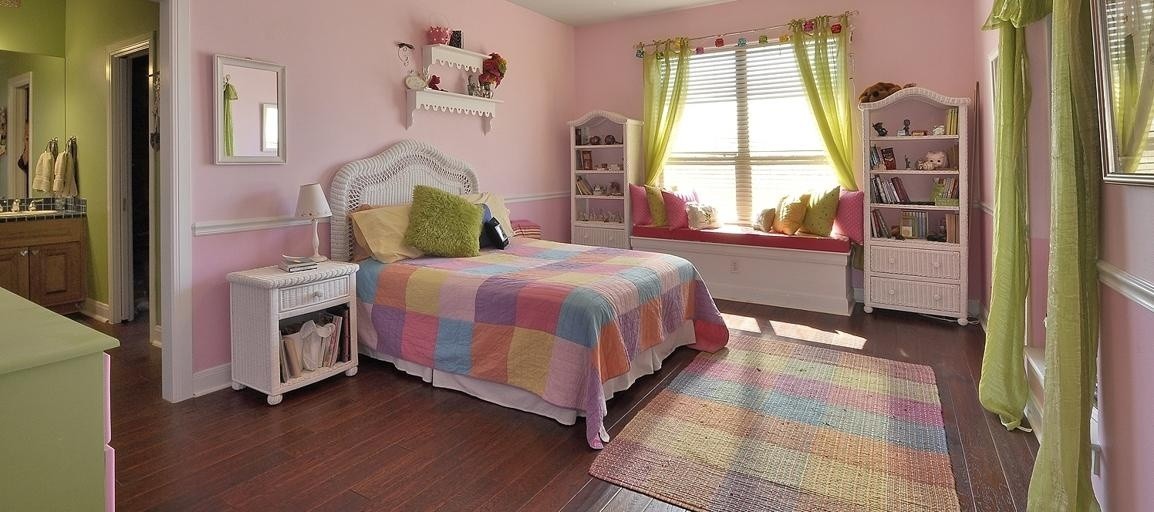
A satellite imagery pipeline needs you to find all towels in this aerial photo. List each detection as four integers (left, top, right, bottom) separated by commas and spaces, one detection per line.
53, 151, 78, 199
32, 152, 54, 193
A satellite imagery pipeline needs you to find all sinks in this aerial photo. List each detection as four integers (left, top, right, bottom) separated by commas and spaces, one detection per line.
0, 210, 56, 216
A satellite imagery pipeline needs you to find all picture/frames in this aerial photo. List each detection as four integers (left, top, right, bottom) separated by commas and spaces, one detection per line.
262, 103, 278, 152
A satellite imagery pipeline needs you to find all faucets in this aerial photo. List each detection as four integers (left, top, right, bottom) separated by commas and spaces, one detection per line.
11, 199, 20, 211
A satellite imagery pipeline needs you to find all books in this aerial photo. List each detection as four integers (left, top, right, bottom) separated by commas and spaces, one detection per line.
900, 210, 929, 239
934, 176, 959, 206
576, 127, 585, 145
945, 107, 959, 135
870, 209, 890, 238
277, 254, 318, 273
945, 213, 960, 244
278, 306, 349, 383
577, 150, 593, 169
871, 173, 911, 204
870, 145, 895, 170
576, 176, 593, 196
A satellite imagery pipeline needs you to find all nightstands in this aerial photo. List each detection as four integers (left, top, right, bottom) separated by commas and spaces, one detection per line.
226, 261, 360, 405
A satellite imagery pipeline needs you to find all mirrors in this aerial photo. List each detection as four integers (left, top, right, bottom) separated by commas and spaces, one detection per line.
212, 53, 287, 166
0, 49, 66, 200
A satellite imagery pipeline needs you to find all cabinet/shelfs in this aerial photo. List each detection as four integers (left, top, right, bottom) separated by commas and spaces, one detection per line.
857, 86, 981, 326
566, 110, 644, 249
0, 286, 120, 512
406, 43, 503, 136
0, 216, 87, 316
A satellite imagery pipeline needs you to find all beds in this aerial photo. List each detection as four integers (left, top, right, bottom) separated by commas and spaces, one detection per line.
329, 140, 728, 450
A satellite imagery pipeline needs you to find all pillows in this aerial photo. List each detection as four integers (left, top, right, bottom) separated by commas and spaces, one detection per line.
349, 186, 515, 262
629, 182, 720, 232
753, 185, 864, 245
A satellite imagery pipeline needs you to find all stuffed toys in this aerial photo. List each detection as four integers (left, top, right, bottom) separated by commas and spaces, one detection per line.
858, 81, 902, 104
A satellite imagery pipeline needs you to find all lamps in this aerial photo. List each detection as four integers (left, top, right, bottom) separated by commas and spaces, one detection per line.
295, 183, 333, 263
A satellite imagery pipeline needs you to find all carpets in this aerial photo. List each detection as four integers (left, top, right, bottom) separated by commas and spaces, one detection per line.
589, 334, 961, 512
